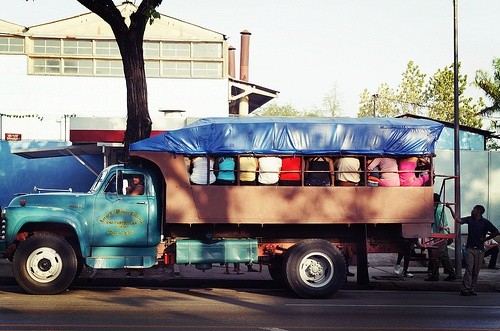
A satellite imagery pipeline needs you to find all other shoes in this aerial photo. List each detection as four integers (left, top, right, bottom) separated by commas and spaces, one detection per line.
138, 272, 145, 278
248, 268, 258, 273
394, 264, 401, 275
402, 270, 413, 278
470, 292, 477, 296
460, 291, 473, 296
126, 272, 131, 278
347, 271, 355, 276
442, 275, 457, 281
487, 265, 499, 270
424, 275, 439, 281
425, 269, 432, 274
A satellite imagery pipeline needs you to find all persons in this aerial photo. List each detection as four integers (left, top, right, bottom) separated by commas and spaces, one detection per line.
126, 175, 144, 195
483, 232, 499, 269
424, 193, 457, 281
446, 202, 500, 297
393, 238, 415, 278
223, 262, 259, 275
190, 155, 430, 186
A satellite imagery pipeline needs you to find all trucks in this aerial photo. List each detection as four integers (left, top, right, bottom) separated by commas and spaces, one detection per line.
0, 115, 459, 299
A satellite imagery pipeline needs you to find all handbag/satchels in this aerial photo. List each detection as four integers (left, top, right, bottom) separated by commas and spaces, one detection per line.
443, 228, 453, 245
